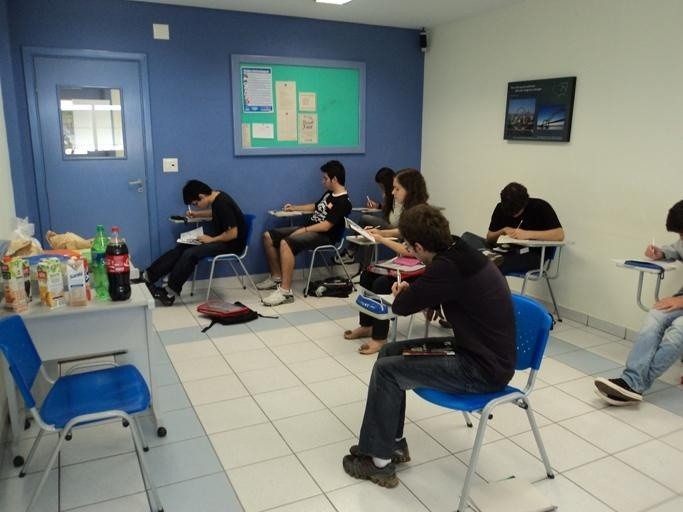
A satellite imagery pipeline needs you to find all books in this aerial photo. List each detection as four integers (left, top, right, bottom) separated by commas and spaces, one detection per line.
401, 340, 456, 357
344, 216, 376, 243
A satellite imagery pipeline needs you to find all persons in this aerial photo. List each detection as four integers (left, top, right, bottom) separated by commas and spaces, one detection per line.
343, 204, 517, 489
331, 167, 402, 284
130, 178, 247, 306
439, 182, 564, 329
256, 159, 352, 307
593, 199, 683, 408
343, 166, 430, 355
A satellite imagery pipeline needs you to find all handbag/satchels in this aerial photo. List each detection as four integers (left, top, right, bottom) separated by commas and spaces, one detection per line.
197, 301, 259, 325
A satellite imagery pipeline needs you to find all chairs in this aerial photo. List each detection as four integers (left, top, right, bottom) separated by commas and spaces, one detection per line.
354, 295, 555, 512
0, 315, 166, 512
170, 214, 263, 303
267, 209, 357, 297
501, 237, 562, 322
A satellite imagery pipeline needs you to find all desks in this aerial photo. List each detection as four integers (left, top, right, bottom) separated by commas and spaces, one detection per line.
0, 282, 166, 468
615, 258, 676, 311
344, 234, 449, 344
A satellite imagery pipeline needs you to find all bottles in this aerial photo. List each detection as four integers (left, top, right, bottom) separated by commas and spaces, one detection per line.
88, 223, 111, 300
103, 225, 132, 302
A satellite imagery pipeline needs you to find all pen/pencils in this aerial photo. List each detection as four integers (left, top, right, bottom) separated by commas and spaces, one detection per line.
188, 204, 192, 218
374, 225, 381, 229
517, 220, 523, 229
396, 269, 401, 291
366, 195, 372, 207
652, 238, 655, 254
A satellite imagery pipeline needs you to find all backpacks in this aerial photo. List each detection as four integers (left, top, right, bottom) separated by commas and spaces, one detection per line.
304, 276, 353, 298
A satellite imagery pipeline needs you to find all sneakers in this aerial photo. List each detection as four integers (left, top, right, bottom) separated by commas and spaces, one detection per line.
359, 342, 386, 355
594, 376, 644, 409
262, 288, 293, 305
349, 437, 411, 463
130, 270, 175, 307
488, 253, 503, 267
344, 326, 372, 338
257, 275, 282, 290
336, 251, 356, 264
343, 454, 398, 488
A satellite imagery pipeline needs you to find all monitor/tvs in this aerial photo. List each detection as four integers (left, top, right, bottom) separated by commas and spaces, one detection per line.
504, 76, 576, 142
